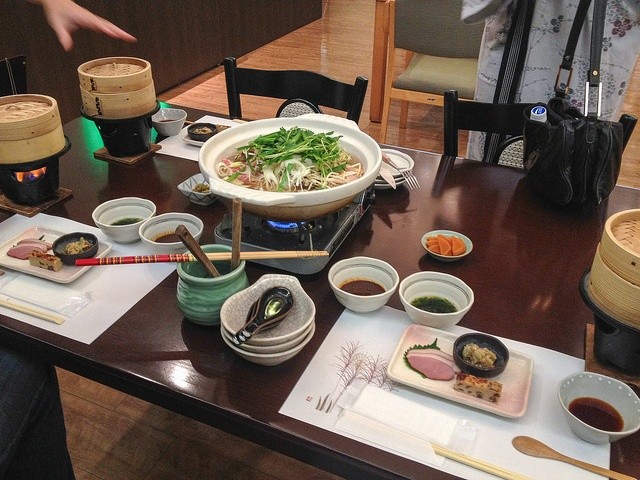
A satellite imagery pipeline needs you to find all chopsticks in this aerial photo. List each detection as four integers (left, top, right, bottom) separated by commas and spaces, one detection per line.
76, 249, 330, 265
431, 441, 532, 480
1, 299, 66, 325
175, 198, 241, 276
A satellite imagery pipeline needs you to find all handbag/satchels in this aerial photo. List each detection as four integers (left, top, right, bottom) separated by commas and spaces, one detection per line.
524, 0, 625, 225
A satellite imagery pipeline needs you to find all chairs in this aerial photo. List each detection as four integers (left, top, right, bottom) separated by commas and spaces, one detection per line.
443, 90, 637, 167
224, 57, 368, 124
378, 0, 486, 143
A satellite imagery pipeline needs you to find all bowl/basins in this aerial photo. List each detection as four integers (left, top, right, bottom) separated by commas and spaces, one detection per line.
188, 122, 217, 141
221, 323, 315, 353
176, 172, 219, 207
557, 372, 640, 444
397, 272, 474, 329
328, 255, 399, 313
91, 196, 157, 244
52, 232, 98, 265
220, 325, 317, 367
420, 229, 473, 263
152, 109, 187, 136
138, 212, 204, 254
219, 274, 316, 345
452, 333, 509, 379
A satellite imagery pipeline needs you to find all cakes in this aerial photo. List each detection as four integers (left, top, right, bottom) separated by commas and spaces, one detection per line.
28, 248, 62, 273
453, 372, 502, 403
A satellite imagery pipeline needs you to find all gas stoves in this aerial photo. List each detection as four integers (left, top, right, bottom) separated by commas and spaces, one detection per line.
214, 188, 378, 275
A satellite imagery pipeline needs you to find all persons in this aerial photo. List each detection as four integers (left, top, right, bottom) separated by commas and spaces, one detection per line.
30, 0, 138, 54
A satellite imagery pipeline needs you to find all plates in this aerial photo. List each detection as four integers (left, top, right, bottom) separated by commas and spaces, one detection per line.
180, 120, 232, 146
375, 181, 404, 189
386, 324, 533, 419
375, 177, 405, 183
375, 147, 414, 177
0, 226, 112, 283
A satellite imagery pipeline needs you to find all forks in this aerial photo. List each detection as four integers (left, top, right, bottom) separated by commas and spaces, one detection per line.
382, 150, 420, 190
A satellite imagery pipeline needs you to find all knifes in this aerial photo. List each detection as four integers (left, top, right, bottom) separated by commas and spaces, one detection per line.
378, 165, 397, 190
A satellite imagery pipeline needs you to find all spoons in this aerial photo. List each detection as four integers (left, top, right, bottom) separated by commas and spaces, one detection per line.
233, 302, 280, 345
241, 287, 294, 335
237, 299, 286, 342
513, 437, 637, 480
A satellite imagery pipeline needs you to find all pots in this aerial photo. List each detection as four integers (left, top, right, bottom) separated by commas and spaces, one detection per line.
198, 113, 383, 221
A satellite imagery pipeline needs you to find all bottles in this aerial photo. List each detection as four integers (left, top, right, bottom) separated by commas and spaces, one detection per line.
529, 106, 553, 125
